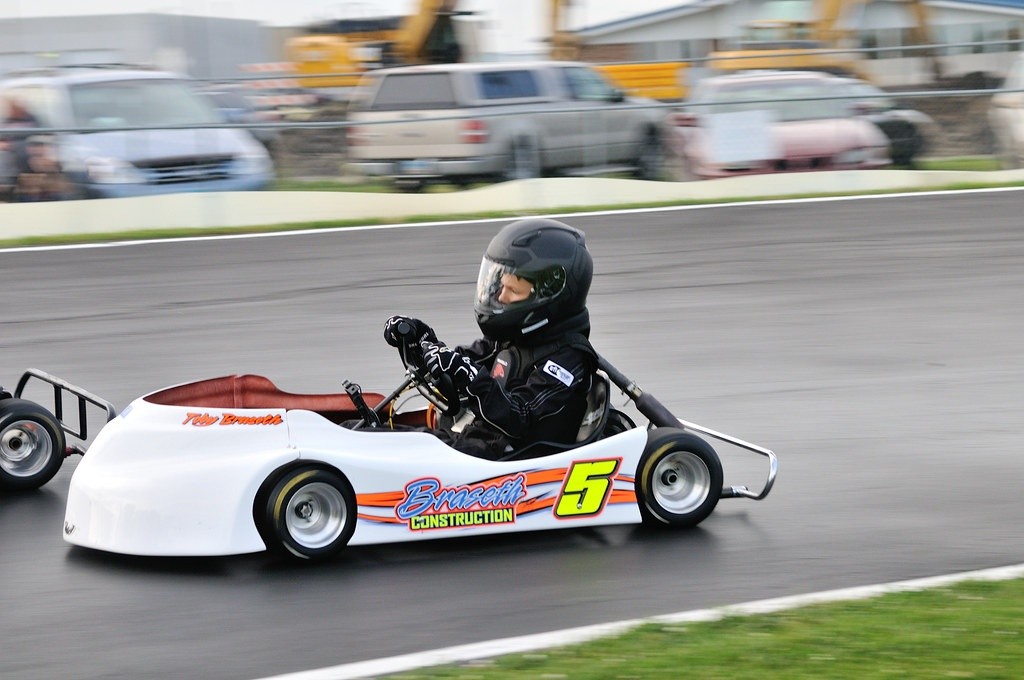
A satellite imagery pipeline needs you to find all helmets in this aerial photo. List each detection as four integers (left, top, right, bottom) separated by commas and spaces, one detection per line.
474, 218, 593, 342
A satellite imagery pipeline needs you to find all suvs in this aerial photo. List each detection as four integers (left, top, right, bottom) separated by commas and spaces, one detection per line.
345, 57, 677, 187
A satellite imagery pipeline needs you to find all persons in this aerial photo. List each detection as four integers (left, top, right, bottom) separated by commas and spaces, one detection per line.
384, 216, 592, 464
0, 99, 42, 175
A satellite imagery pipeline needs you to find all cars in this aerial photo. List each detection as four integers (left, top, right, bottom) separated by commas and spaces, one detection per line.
1, 72, 277, 203
833, 71, 939, 167
667, 72, 895, 177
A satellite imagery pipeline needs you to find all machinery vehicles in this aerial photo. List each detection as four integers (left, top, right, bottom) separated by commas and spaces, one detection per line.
284, 1, 578, 106
702, 0, 946, 75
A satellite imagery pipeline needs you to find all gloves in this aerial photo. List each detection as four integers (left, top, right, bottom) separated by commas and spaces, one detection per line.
383, 315, 439, 367
421, 341, 479, 389
